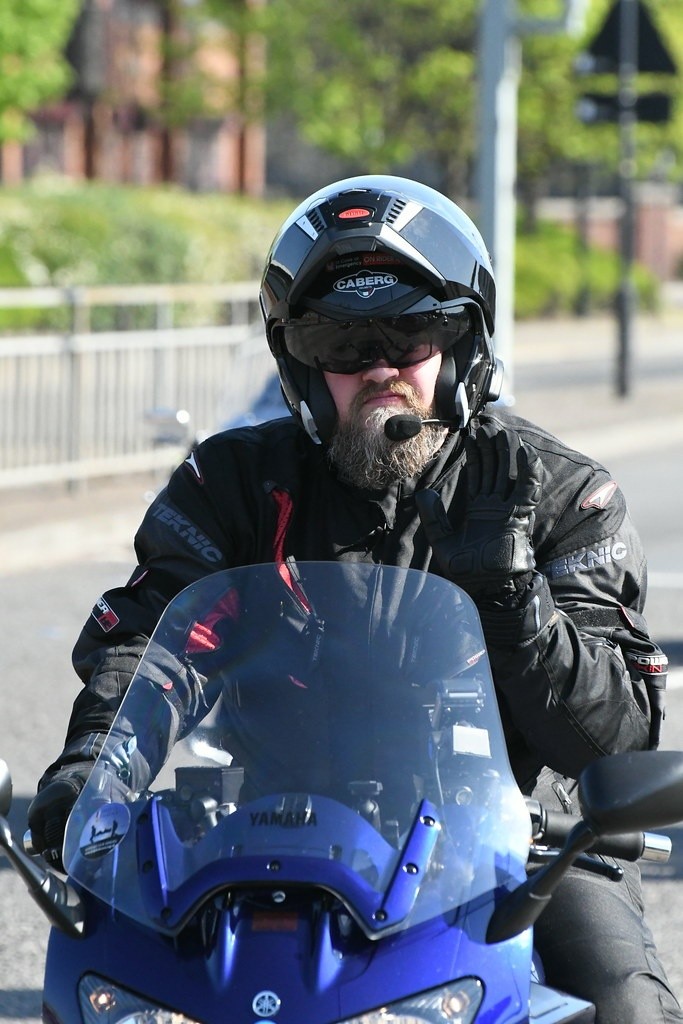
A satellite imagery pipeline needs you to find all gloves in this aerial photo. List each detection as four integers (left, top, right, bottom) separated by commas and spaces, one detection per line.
30, 760, 136, 875
419, 425, 545, 592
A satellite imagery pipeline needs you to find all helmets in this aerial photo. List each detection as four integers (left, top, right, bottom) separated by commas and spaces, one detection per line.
259, 171, 498, 453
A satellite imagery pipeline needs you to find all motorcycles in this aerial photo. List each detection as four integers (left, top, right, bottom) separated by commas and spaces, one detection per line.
3, 559, 680, 1021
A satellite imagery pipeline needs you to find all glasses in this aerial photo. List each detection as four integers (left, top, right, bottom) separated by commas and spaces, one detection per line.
273, 309, 473, 373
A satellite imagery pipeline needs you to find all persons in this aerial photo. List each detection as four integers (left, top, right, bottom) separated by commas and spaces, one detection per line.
24, 171, 683, 1024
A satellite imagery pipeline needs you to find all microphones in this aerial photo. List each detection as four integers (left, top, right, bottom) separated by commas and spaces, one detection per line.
384, 414, 456, 442
